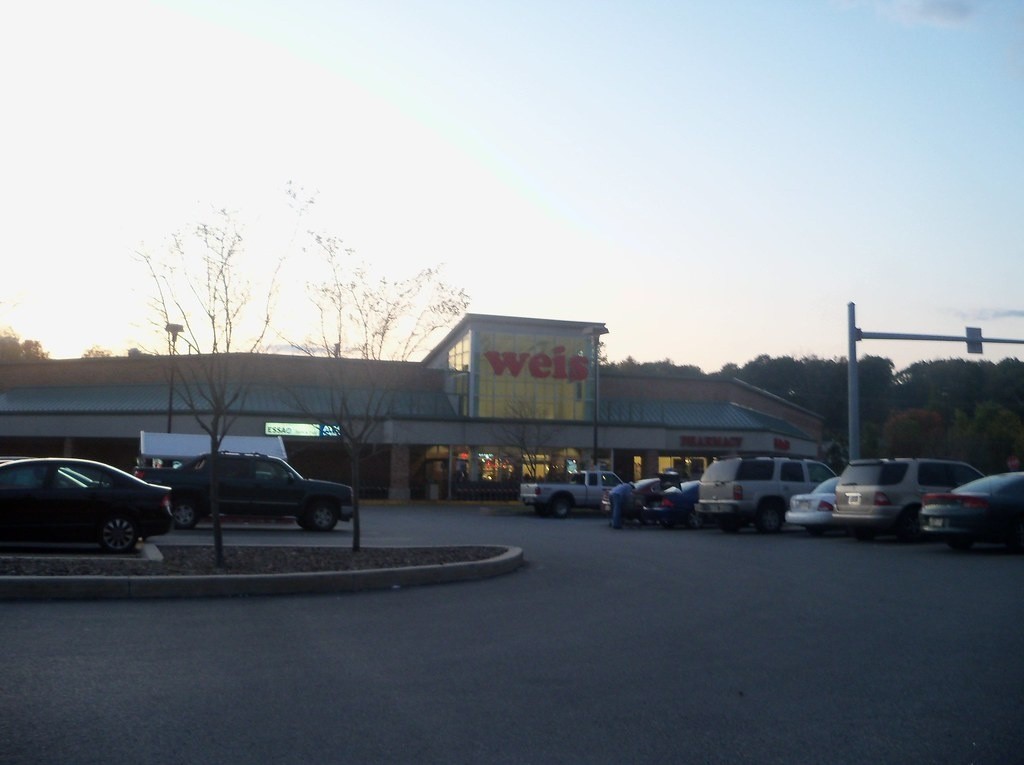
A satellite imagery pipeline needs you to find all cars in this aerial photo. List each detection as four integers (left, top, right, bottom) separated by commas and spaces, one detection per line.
131, 447, 357, 534
600, 477, 664, 524
918, 471, 1024, 551
640, 479, 704, 527
0, 454, 174, 553
784, 476, 840, 537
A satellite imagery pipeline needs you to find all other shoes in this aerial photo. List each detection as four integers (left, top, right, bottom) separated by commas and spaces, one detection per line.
613, 525, 623, 530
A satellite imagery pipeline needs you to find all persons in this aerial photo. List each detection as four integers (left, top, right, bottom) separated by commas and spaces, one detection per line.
608, 482, 635, 529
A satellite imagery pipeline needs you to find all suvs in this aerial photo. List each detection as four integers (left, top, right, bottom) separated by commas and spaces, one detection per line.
830, 457, 989, 543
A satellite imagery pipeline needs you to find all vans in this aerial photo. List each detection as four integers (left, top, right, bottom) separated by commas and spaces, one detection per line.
692, 455, 843, 533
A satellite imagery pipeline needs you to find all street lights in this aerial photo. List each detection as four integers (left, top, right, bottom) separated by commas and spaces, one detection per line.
582, 326, 609, 467
164, 323, 185, 437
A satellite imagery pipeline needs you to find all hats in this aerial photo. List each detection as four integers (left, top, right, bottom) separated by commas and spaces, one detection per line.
629, 481, 636, 489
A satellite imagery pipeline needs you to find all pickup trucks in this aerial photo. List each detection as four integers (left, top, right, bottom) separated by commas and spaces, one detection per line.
519, 470, 633, 517
